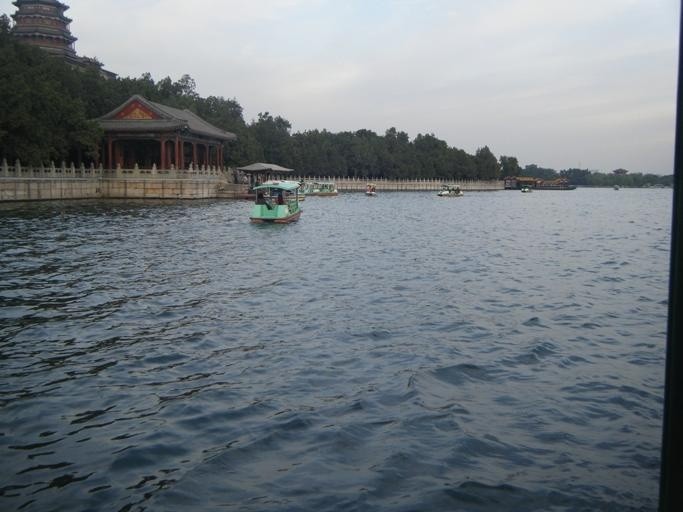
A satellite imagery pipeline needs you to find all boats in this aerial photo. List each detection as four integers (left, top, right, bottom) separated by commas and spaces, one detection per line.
521, 185, 532, 192
237, 163, 338, 224
366, 191, 377, 196
438, 184, 463, 197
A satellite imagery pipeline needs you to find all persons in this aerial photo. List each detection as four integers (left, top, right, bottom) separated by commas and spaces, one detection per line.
366, 184, 376, 192
257, 190, 272, 209
444, 186, 460, 194
277, 190, 283, 205
